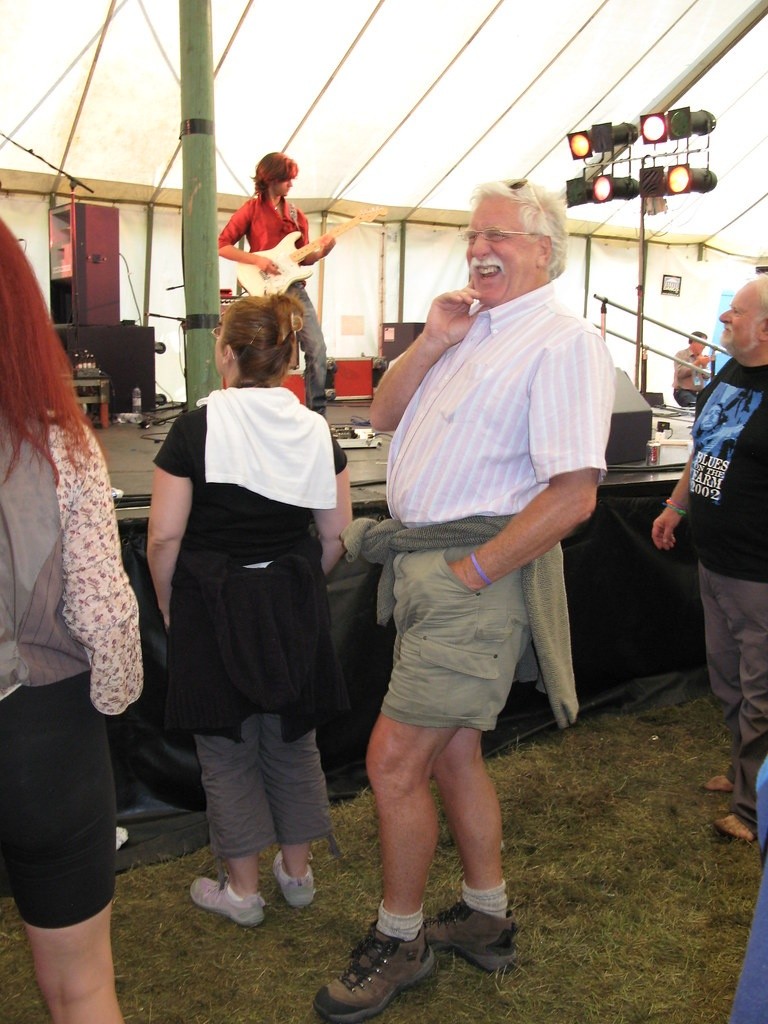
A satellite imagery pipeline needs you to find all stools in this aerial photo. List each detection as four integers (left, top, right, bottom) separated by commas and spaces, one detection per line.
68, 376, 110, 429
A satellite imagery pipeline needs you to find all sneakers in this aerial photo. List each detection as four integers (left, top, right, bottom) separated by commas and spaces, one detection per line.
190, 877, 265, 927
312, 919, 440, 1024
273, 850, 313, 906
424, 899, 518, 970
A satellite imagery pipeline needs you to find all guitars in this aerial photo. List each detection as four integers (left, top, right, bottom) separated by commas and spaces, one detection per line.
239, 206, 387, 303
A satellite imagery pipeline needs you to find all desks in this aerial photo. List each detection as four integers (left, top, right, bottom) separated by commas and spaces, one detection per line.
53, 325, 157, 416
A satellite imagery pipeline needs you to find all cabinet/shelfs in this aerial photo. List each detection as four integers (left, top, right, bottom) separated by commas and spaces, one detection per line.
47, 201, 122, 326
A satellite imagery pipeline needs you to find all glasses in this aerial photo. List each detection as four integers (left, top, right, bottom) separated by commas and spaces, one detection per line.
458, 228, 535, 241
501, 179, 544, 215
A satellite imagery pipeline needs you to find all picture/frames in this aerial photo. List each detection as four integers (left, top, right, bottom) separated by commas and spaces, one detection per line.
661, 275, 682, 296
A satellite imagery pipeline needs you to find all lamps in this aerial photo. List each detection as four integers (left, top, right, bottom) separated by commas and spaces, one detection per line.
668, 106, 717, 141
591, 121, 639, 154
640, 113, 669, 145
592, 173, 641, 205
566, 177, 592, 207
566, 130, 594, 161
639, 165, 667, 199
667, 163, 719, 195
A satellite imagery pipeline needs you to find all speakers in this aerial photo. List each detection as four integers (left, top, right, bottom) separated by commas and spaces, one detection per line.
54, 323, 157, 415
604, 367, 653, 464
48, 202, 121, 326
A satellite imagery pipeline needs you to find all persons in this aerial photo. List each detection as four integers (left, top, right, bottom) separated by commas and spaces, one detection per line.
219, 152, 336, 417
147, 294, 353, 928
672, 331, 711, 407
312, 179, 615, 1024
652, 274, 768, 843
0, 220, 144, 1024
729, 755, 768, 1024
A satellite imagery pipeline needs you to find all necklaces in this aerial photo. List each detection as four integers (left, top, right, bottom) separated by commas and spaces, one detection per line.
267, 195, 282, 210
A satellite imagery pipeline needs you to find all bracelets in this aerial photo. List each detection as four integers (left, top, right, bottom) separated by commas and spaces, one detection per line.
470, 553, 494, 585
663, 500, 688, 516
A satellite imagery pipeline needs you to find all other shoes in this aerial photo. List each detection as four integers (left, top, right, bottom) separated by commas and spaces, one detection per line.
712, 815, 755, 842
702, 776, 734, 792
